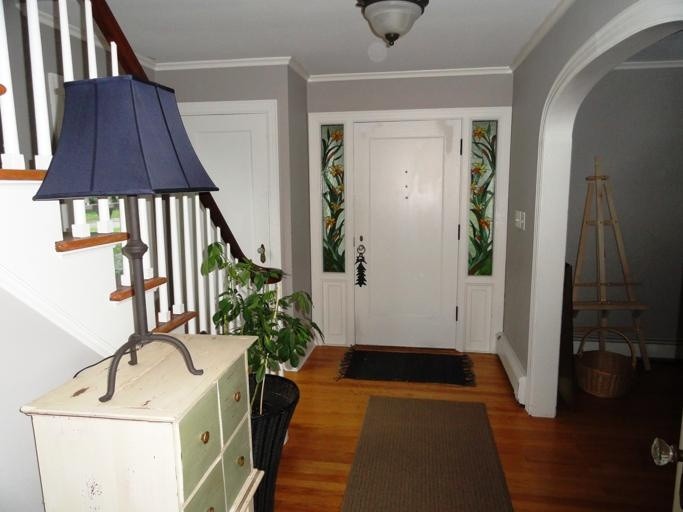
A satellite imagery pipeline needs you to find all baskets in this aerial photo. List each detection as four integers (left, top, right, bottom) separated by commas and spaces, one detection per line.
573, 325, 637, 401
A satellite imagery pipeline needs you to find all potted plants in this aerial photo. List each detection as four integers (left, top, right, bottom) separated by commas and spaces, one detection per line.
201, 241, 325, 512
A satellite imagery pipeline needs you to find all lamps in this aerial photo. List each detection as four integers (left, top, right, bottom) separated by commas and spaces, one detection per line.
355, 0, 431, 47
32, 76, 220, 403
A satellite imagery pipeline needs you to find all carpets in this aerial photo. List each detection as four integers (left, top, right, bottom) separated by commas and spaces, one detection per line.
339, 395, 514, 512
334, 343, 476, 387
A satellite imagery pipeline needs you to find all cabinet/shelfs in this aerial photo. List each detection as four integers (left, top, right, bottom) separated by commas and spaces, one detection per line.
20, 332, 266, 512
